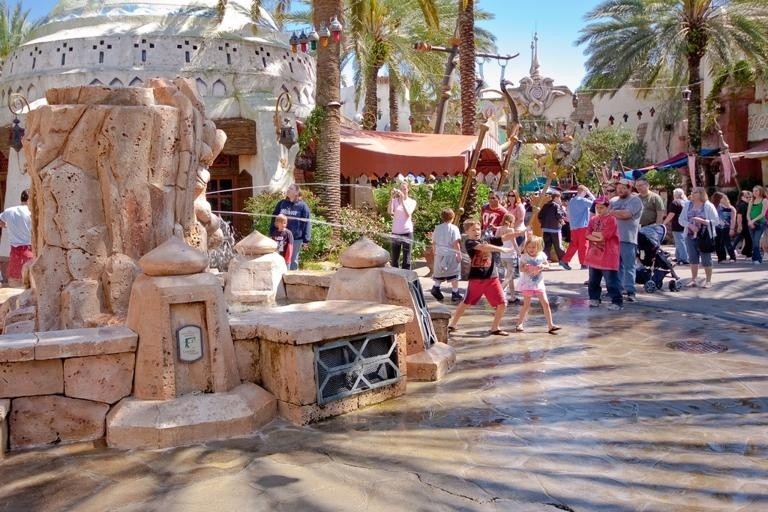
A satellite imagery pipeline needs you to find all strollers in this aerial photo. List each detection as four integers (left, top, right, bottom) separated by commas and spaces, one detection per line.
636, 223, 683, 293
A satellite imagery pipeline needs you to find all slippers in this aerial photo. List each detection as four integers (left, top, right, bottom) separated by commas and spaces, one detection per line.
489, 330, 509, 336
516, 325, 523, 331
549, 326, 560, 332
448, 327, 458, 333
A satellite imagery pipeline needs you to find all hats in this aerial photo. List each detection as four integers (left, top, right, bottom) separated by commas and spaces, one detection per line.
614, 179, 632, 186
596, 197, 609, 205
553, 191, 560, 194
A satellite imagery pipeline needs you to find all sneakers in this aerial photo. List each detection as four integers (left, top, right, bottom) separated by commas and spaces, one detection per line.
559, 260, 571, 270
684, 280, 696, 287
702, 282, 711, 288
452, 290, 463, 304
608, 304, 624, 311
601, 293, 613, 301
431, 286, 444, 300
581, 264, 589, 269
589, 299, 600, 306
627, 292, 635, 301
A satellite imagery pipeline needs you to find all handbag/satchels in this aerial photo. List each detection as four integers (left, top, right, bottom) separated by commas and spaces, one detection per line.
697, 225, 722, 252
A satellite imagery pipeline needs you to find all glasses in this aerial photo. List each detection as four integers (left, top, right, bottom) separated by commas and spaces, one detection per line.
635, 183, 647, 188
507, 194, 515, 197
488, 196, 496, 200
607, 189, 615, 192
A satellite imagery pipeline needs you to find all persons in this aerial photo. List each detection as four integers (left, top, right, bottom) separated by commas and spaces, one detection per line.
388, 183, 417, 270
746, 185, 768, 264
559, 184, 596, 270
521, 192, 551, 208
430, 209, 463, 302
662, 188, 689, 267
478, 191, 510, 285
607, 180, 619, 199
272, 184, 312, 270
560, 189, 575, 242
594, 180, 610, 205
272, 214, 294, 270
635, 177, 666, 228
447, 219, 516, 336
608, 178, 643, 302
0, 189, 33, 280
515, 235, 561, 332
737, 190, 755, 261
538, 191, 566, 260
711, 192, 732, 265
732, 190, 746, 251
496, 213, 522, 302
584, 197, 624, 310
721, 194, 738, 263
678, 186, 721, 288
759, 184, 767, 260
505, 189, 526, 278
